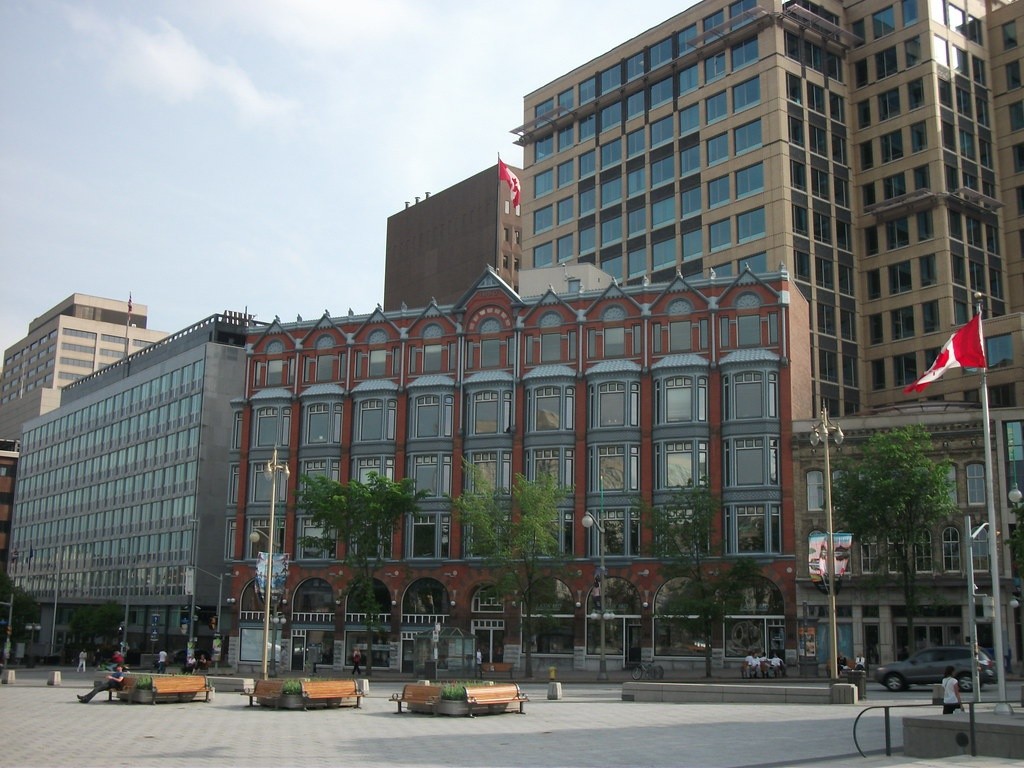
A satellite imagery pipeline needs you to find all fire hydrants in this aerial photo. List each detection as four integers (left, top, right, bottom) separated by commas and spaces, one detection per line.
549, 666, 557, 680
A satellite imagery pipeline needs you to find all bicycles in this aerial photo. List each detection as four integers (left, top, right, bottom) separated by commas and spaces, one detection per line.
631, 660, 664, 680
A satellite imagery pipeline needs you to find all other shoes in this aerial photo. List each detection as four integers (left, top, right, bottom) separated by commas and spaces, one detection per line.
77, 695, 89, 703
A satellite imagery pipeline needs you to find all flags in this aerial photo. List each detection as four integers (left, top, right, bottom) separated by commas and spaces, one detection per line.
500, 159, 521, 208
902, 311, 988, 397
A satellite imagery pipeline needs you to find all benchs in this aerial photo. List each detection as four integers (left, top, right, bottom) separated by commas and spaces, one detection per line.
300, 678, 363, 711
149, 674, 212, 705
480, 662, 515, 679
240, 679, 285, 709
389, 683, 442, 717
109, 676, 138, 704
464, 682, 529, 719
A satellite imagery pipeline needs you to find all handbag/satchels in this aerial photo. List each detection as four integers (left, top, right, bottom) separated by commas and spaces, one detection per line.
108, 678, 125, 691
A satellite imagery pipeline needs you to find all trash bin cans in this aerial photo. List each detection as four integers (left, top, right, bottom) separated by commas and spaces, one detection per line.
846, 669, 868, 701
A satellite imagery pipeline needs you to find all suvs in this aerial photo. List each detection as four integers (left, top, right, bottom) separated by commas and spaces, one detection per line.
873, 643, 998, 692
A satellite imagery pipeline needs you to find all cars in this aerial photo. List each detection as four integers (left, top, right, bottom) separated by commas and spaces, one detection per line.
168, 648, 211, 665
48, 644, 151, 666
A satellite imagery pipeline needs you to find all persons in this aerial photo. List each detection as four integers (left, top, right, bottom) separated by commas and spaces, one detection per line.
743, 649, 789, 680
838, 651, 848, 674
91, 648, 102, 668
182, 653, 206, 675
77, 647, 88, 672
151, 647, 168, 674
473, 648, 484, 680
107, 651, 130, 673
348, 645, 367, 675
855, 653, 865, 672
77, 663, 124, 704
942, 666, 965, 715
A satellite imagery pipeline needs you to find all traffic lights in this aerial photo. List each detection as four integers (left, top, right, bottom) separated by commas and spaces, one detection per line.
988, 531, 1000, 555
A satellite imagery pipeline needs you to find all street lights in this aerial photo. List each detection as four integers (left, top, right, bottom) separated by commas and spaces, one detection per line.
249, 449, 290, 680
581, 475, 616, 682
1009, 449, 1024, 677
189, 518, 200, 642
25, 622, 42, 669
186, 566, 223, 669
809, 399, 844, 679
250, 502, 287, 678
